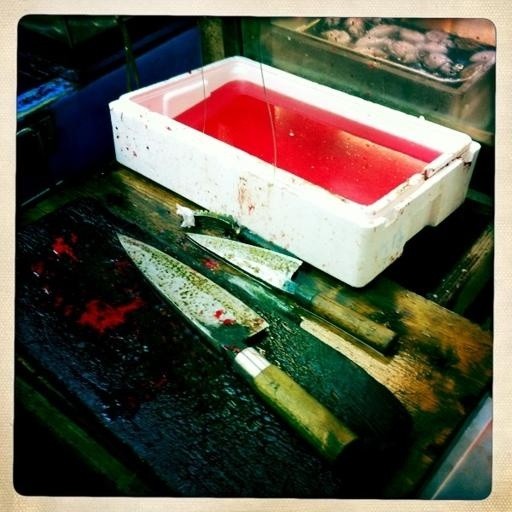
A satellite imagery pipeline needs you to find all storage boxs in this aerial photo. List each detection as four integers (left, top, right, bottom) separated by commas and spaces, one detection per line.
108, 55, 483, 289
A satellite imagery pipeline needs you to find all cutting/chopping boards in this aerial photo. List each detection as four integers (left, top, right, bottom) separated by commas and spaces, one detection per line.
14, 169, 484, 499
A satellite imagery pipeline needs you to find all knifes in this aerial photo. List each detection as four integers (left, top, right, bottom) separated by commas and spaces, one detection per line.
186, 233, 400, 359
116, 232, 365, 468
